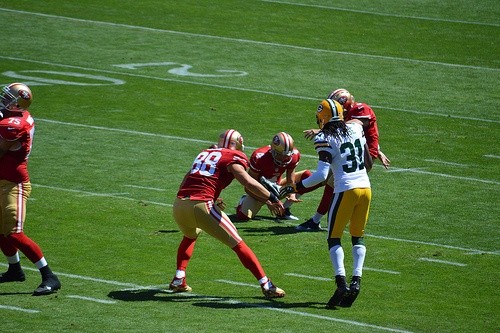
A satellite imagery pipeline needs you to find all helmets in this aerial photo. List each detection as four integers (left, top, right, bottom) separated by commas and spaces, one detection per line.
0, 82, 32, 112
328, 88, 352, 112
217, 129, 245, 152
316, 98, 344, 129
271, 131, 294, 167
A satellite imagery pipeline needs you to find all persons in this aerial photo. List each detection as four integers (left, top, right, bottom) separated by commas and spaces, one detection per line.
278, 99, 373, 309
169, 129, 286, 298
235, 88, 391, 231
0, 83, 61, 295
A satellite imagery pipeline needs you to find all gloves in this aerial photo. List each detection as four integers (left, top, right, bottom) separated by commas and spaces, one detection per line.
377, 152, 391, 171
268, 193, 285, 216
278, 182, 304, 201
215, 197, 226, 211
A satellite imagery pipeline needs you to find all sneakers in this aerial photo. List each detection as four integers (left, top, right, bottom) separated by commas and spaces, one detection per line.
34, 274, 61, 296
327, 285, 350, 306
277, 207, 299, 221
0, 270, 26, 283
238, 195, 248, 206
260, 279, 286, 297
297, 218, 321, 230
168, 276, 192, 293
339, 280, 361, 307
